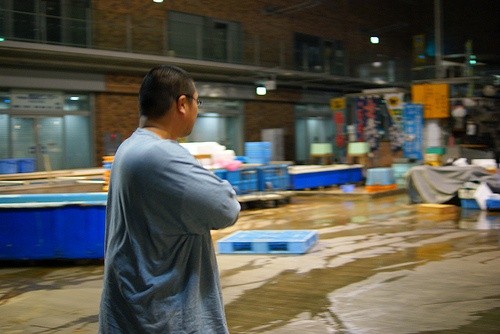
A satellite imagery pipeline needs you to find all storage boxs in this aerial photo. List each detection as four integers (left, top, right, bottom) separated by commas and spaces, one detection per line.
0, 158, 35, 175
458, 182, 500, 211
391, 146, 446, 188
212, 128, 291, 196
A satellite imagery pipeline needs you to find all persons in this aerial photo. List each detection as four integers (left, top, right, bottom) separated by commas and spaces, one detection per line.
98, 64, 240, 334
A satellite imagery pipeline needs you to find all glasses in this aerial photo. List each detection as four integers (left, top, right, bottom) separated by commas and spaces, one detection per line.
175, 94, 202, 107
